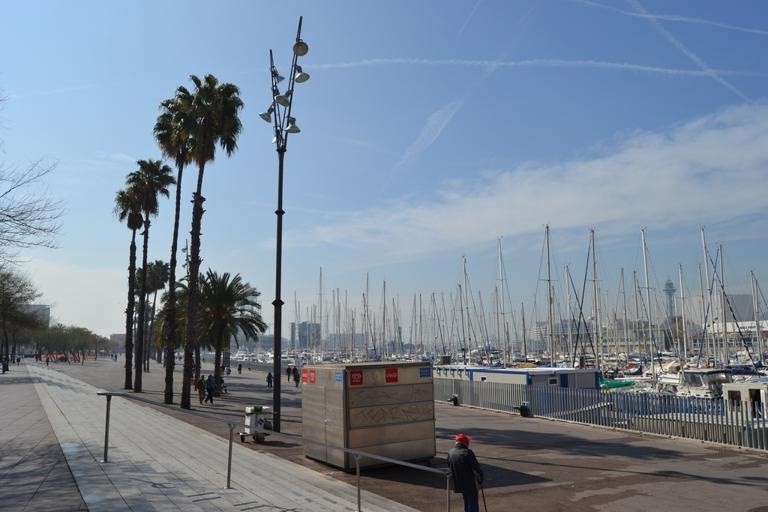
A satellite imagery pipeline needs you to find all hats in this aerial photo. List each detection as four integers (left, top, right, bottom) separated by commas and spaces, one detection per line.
456, 432, 472, 441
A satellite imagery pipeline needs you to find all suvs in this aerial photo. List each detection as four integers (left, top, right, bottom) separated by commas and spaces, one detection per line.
2, 352, 9, 362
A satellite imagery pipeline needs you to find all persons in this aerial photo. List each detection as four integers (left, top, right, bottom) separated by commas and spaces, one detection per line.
446, 432, 483, 512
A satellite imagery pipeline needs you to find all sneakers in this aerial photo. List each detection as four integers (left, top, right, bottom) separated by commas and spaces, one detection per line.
200, 401, 213, 404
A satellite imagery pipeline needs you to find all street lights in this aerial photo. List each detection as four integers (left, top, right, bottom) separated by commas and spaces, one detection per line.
261, 15, 310, 432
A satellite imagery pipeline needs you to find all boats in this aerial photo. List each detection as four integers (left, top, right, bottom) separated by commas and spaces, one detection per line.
173, 351, 222, 359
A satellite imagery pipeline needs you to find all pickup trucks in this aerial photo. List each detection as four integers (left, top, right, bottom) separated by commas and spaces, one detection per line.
23, 351, 38, 358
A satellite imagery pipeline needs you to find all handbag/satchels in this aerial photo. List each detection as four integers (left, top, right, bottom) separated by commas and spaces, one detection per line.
453, 470, 474, 493
